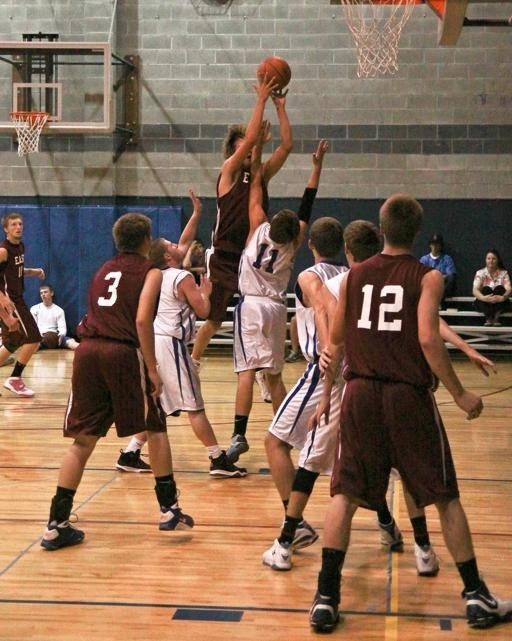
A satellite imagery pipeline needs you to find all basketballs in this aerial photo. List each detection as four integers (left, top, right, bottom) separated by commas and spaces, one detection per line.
258, 56, 291, 92
43, 332, 60, 349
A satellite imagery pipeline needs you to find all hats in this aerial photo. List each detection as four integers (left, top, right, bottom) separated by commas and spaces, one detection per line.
429, 233, 444, 243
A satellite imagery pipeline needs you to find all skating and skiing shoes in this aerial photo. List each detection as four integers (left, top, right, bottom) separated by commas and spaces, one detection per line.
40, 519, 85, 551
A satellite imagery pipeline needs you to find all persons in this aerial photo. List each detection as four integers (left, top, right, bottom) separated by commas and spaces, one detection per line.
307, 192, 512, 631
41, 213, 194, 551
263, 216, 405, 552
1, 213, 46, 397
227, 119, 332, 470
261, 219, 498, 572
190, 72, 293, 404
30, 285, 81, 350
472, 251, 511, 326
418, 233, 457, 311
116, 188, 247, 477
182, 239, 207, 279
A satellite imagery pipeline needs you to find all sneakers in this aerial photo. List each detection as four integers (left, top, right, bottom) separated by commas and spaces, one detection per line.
291, 519, 319, 550
254, 371, 272, 404
261, 538, 292, 570
413, 543, 439, 576
189, 354, 200, 374
115, 449, 153, 473
378, 519, 403, 549
3, 376, 35, 398
158, 502, 194, 532
208, 450, 248, 477
225, 433, 249, 463
461, 581, 512, 629
307, 591, 339, 634
